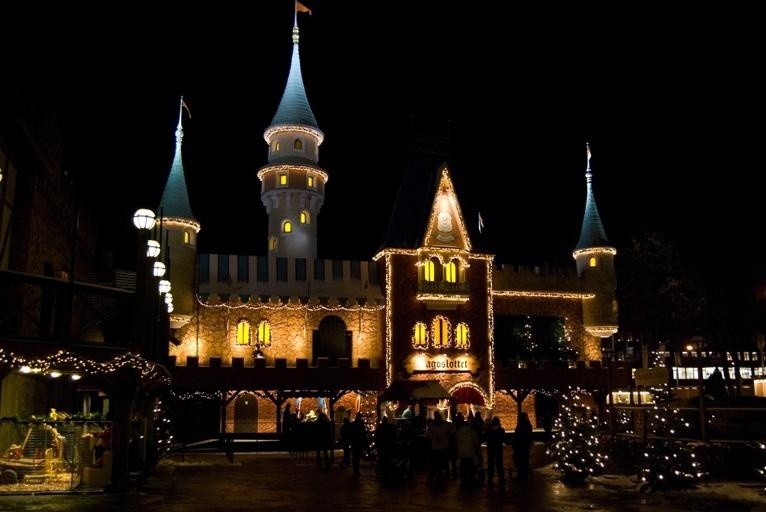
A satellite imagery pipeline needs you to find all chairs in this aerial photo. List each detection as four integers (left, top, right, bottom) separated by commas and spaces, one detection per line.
82, 452, 112, 486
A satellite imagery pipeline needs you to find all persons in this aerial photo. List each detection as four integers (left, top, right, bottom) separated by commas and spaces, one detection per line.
95, 424, 112, 466
282, 404, 533, 497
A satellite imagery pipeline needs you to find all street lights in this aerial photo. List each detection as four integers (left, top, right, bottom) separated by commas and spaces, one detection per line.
132, 208, 174, 363
